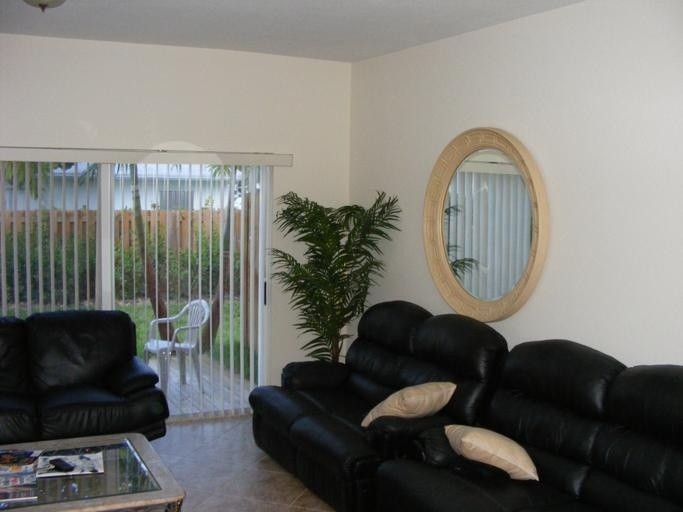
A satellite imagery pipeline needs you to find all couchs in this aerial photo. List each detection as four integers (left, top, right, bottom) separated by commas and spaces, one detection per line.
249, 301, 508, 512
0, 310, 169, 442
373, 340, 683, 512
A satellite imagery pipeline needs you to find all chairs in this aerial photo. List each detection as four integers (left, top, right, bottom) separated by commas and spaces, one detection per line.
144, 300, 209, 398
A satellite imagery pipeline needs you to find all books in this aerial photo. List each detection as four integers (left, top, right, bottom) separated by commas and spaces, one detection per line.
0, 448, 104, 504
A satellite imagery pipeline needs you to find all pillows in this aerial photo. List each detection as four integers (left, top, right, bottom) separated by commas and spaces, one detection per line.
360, 382, 458, 428
444, 424, 540, 481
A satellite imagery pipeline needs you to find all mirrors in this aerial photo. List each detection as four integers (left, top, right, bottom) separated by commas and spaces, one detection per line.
423, 128, 550, 323
